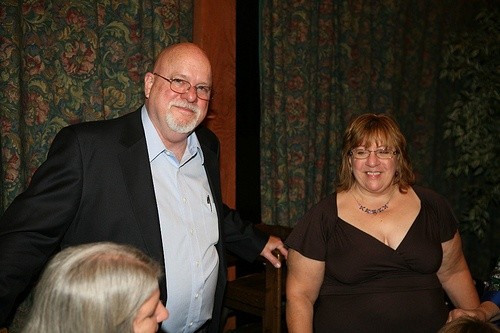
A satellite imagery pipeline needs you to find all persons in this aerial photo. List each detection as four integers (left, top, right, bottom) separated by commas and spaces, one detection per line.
17, 243, 168, 333
286, 114, 500, 333
0, 43, 288, 333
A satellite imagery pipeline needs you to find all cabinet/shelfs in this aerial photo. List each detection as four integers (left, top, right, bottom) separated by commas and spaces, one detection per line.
223, 223, 293, 333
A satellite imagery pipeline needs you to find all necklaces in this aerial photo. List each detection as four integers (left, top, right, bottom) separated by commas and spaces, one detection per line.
349, 190, 396, 214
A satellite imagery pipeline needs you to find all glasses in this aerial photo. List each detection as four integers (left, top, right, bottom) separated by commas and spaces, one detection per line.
349, 149, 397, 159
153, 73, 214, 101
